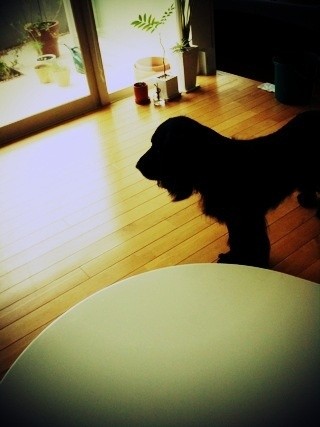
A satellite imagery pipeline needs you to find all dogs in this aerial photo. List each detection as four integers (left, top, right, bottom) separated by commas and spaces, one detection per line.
135, 107, 320, 267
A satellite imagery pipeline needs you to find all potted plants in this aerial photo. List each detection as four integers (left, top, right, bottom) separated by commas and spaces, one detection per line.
32, 41, 56, 72
131, 2, 181, 103
24, 0, 63, 59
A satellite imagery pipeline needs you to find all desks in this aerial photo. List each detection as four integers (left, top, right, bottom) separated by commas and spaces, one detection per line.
0, 262, 320, 427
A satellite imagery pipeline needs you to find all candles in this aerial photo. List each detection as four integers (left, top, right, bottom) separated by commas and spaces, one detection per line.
170, 0, 201, 93
